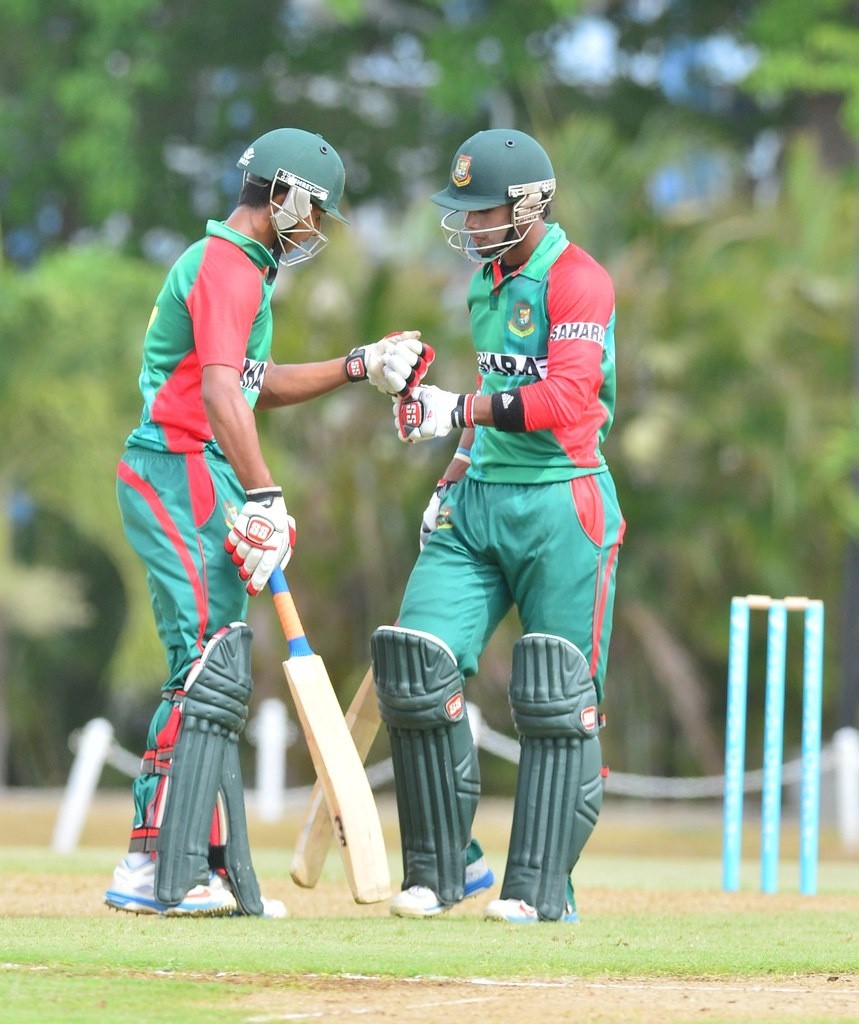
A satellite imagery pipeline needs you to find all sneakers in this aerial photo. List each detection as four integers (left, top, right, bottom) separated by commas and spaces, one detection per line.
106, 853, 238, 918
486, 871, 580, 923
388, 839, 494, 920
208, 867, 289, 918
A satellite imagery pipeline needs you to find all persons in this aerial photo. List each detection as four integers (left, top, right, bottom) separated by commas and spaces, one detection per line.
104, 126, 437, 918
372, 128, 624, 925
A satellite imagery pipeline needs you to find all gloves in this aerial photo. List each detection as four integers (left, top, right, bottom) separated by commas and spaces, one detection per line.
420, 479, 456, 554
392, 383, 477, 444
224, 485, 297, 596
346, 330, 435, 400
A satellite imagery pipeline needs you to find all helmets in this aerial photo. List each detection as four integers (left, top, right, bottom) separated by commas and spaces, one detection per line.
236, 128, 352, 267
430, 128, 557, 264
